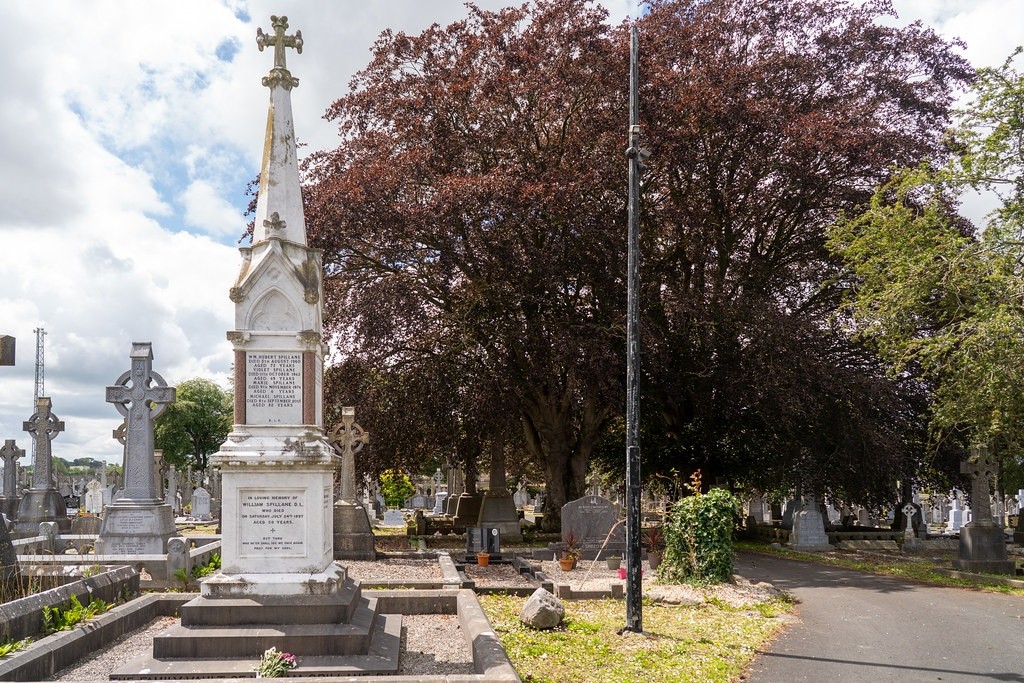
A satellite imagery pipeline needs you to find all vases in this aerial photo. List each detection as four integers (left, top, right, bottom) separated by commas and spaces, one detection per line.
616, 569, 645, 579
605, 555, 621, 570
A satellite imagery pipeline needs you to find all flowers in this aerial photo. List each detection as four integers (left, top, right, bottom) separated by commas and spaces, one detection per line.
254, 646, 297, 678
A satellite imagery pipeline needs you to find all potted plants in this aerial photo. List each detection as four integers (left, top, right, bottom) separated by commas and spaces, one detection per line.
641, 526, 664, 569
477, 549, 490, 566
559, 528, 583, 571
